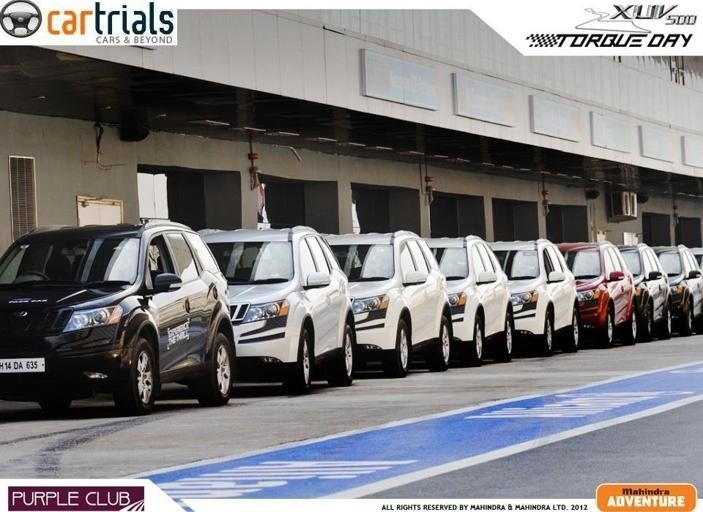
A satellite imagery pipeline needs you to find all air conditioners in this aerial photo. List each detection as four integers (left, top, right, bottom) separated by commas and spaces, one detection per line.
610, 189, 629, 218
629, 191, 637, 217
